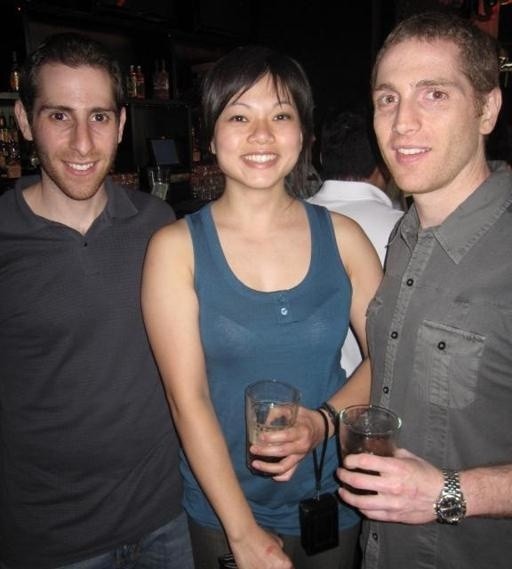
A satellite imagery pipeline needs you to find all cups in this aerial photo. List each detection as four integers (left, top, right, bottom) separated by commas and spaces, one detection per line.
338, 404, 403, 496
243, 380, 302, 478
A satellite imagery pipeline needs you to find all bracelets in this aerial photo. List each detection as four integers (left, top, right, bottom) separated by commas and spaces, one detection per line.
319, 402, 340, 439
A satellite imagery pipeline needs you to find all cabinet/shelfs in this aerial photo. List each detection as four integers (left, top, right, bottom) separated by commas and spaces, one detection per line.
0, 1, 261, 219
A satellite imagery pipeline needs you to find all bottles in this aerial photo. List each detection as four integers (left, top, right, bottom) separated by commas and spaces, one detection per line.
0, 113, 22, 179
126, 59, 171, 101
191, 127, 201, 169
9, 50, 21, 93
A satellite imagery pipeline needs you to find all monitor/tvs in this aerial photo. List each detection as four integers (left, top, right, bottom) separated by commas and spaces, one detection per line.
145, 136, 179, 166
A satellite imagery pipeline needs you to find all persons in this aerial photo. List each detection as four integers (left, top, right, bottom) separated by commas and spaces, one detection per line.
1, 39, 197, 569
297, 115, 407, 380
336, 10, 512, 569
138, 43, 383, 568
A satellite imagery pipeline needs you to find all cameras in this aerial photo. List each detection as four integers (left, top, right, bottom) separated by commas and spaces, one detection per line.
299, 493, 340, 556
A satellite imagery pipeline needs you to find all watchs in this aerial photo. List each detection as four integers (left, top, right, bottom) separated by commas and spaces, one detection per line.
433, 468, 466, 526
